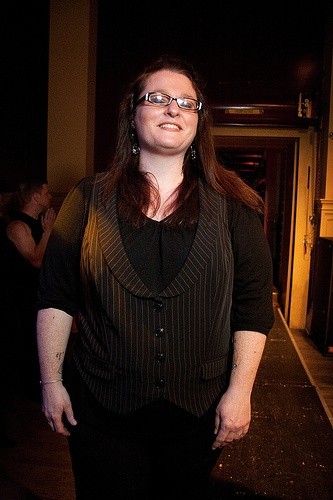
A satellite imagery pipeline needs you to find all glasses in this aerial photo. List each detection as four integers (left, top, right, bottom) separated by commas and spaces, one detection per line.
134, 92, 202, 110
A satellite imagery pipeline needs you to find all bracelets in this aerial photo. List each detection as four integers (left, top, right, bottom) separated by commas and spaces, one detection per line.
40, 378, 63, 385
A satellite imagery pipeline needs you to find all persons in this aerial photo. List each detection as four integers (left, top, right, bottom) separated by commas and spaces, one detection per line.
36, 57, 274, 500
0, 173, 57, 269
